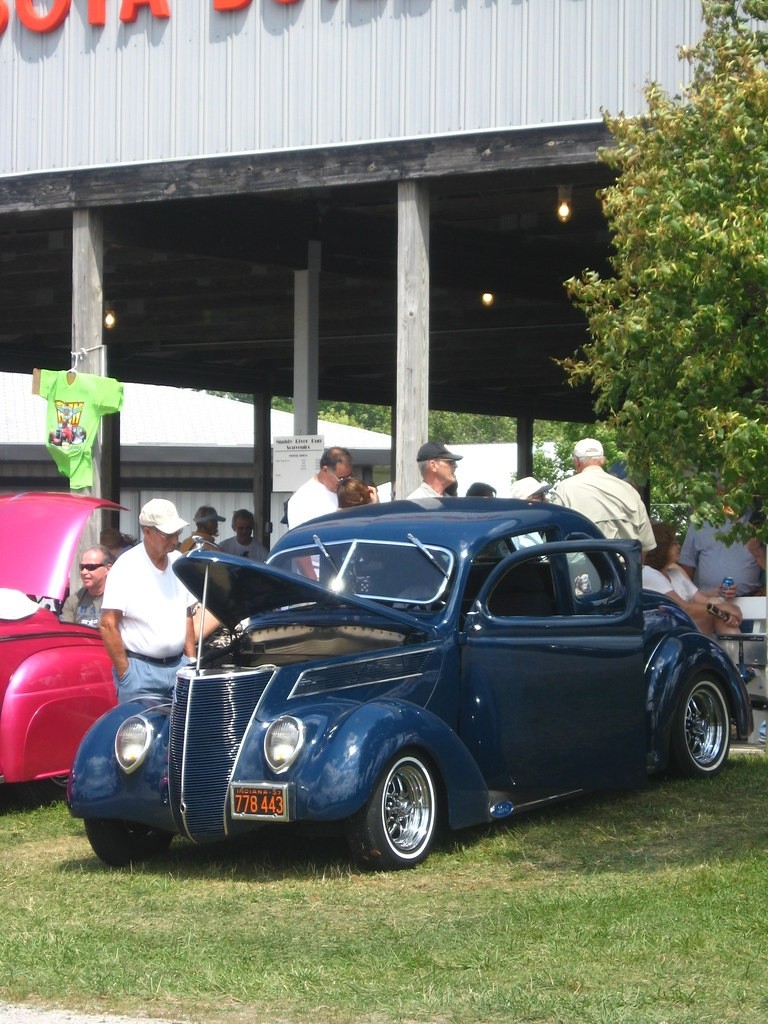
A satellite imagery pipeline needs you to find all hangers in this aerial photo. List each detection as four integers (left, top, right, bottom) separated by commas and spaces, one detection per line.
66, 347, 87, 374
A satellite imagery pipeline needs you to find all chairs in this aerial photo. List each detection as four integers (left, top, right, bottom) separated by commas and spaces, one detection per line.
489, 564, 552, 617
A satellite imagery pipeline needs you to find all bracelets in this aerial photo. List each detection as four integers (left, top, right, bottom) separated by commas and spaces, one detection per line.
189, 657, 197, 663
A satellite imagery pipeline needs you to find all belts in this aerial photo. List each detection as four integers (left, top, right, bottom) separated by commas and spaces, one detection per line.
126, 650, 183, 664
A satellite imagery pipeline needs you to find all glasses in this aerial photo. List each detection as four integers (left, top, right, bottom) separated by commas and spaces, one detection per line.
148, 528, 183, 538
333, 474, 351, 481
237, 527, 252, 530
533, 494, 545, 499
79, 564, 104, 571
672, 541, 680, 545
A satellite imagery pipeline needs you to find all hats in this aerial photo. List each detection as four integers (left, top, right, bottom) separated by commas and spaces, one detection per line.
574, 438, 603, 457
416, 441, 462, 460
512, 477, 553, 500
193, 507, 225, 523
139, 499, 189, 534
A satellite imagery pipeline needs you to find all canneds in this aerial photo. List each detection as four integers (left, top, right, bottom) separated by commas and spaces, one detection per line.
719, 577, 734, 596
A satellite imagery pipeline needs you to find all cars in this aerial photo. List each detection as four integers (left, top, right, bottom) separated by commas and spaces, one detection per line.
66, 493, 753, 871
1, 490, 125, 799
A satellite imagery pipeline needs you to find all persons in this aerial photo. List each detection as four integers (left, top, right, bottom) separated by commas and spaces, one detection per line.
405, 441, 508, 562
285, 446, 380, 594
98, 497, 197, 707
99, 529, 134, 559
641, 524, 755, 685
553, 438, 658, 600
504, 477, 552, 563
217, 508, 281, 611
59, 544, 116, 631
180, 507, 231, 602
677, 474, 767, 634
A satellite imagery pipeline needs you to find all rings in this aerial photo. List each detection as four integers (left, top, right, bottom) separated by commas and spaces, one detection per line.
735, 621, 738, 624
